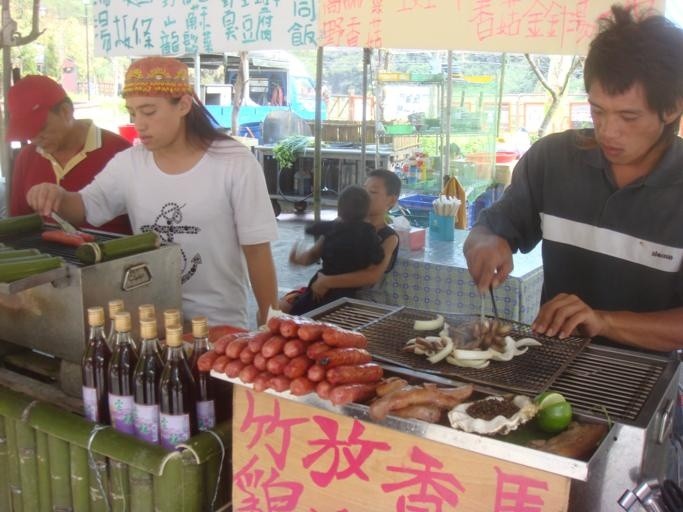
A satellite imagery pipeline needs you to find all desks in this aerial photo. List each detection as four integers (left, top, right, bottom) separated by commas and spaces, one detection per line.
256, 141, 393, 216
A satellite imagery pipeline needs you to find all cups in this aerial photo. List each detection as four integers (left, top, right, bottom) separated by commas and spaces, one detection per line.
428, 213, 455, 242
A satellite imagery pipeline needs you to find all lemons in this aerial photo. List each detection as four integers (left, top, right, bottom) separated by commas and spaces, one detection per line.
531, 389, 574, 435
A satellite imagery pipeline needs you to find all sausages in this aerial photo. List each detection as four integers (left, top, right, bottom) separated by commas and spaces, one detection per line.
41, 228, 98, 248
196, 316, 475, 424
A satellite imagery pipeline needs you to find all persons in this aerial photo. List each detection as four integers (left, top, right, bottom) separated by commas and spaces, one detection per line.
288, 184, 383, 317
3, 73, 134, 236
279, 167, 401, 314
25, 54, 281, 334
461, 3, 681, 354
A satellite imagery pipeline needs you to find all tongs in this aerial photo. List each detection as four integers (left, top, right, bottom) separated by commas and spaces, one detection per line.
478, 273, 501, 334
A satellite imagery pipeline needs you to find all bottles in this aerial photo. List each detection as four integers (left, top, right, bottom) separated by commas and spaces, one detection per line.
394, 151, 434, 184
78, 296, 215, 446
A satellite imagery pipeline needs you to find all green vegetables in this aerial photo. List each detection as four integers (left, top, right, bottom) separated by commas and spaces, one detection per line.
273, 135, 329, 172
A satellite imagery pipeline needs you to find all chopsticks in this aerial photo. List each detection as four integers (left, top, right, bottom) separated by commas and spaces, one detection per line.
431, 196, 462, 215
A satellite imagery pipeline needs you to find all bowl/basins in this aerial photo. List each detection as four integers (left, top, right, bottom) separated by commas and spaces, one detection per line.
466, 150, 518, 163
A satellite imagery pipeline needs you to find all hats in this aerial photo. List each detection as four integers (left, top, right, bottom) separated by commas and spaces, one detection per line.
120, 57, 193, 100
4, 74, 67, 142
